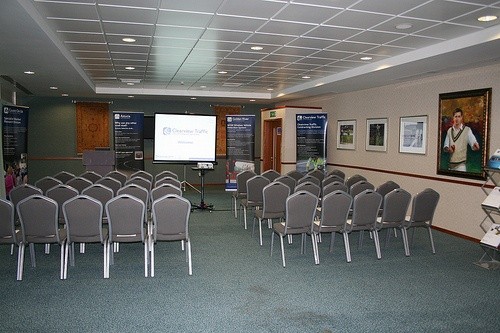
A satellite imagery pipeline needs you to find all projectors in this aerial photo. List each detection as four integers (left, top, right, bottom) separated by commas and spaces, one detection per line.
196, 162, 214, 169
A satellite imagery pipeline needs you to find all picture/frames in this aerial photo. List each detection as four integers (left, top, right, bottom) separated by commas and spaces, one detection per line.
398, 114, 429, 156
336, 119, 357, 150
365, 117, 390, 154
437, 87, 492, 181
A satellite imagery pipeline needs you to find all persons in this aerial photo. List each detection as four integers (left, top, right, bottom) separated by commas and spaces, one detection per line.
443, 108, 479, 171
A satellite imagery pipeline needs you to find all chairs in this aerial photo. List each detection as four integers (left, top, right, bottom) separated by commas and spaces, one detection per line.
0, 169, 192, 281
230, 168, 439, 268
